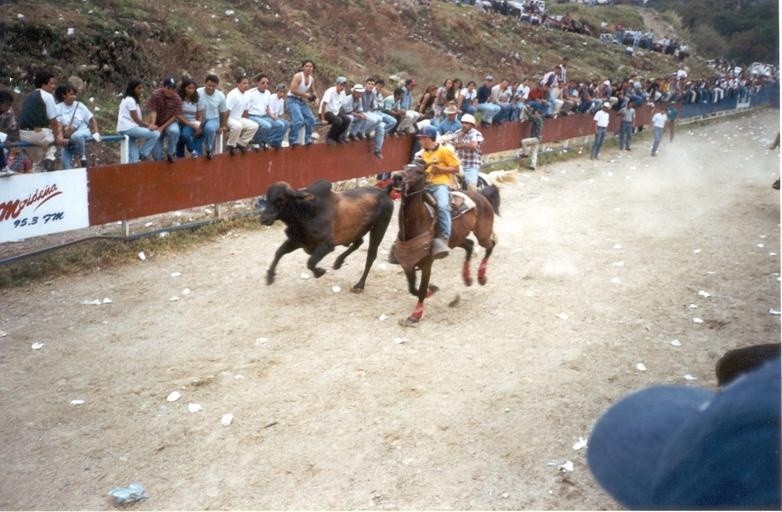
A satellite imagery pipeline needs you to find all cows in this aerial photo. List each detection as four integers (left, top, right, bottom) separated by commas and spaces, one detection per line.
258, 179, 394, 294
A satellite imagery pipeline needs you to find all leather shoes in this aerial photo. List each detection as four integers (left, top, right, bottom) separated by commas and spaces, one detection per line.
81, 160, 88, 167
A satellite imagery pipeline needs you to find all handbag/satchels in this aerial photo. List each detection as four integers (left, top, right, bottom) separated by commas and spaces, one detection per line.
63, 126, 75, 138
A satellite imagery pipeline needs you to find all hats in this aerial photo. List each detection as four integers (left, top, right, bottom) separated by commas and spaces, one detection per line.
461, 113, 476, 125
587, 358, 782, 510
406, 79, 417, 87
276, 83, 286, 92
336, 76, 348, 85
164, 78, 176, 87
351, 84, 366, 93
485, 75, 494, 81
604, 80, 611, 87
442, 105, 463, 115
601, 102, 612, 111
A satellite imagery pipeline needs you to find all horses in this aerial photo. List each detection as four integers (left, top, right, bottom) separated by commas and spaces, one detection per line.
455, 171, 493, 191
391, 159, 503, 326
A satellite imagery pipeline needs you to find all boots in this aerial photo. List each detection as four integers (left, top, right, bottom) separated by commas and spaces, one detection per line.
44, 159, 54, 171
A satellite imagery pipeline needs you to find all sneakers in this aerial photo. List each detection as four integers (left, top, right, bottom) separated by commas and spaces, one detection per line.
140, 155, 146, 161
0, 166, 15, 177
191, 150, 200, 160
480, 120, 502, 128
526, 167, 534, 170
325, 128, 410, 144
226, 141, 313, 156
207, 150, 213, 160
520, 153, 528, 158
168, 153, 176, 163
374, 152, 383, 159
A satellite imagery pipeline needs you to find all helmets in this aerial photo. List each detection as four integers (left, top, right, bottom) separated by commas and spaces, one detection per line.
415, 126, 436, 138
